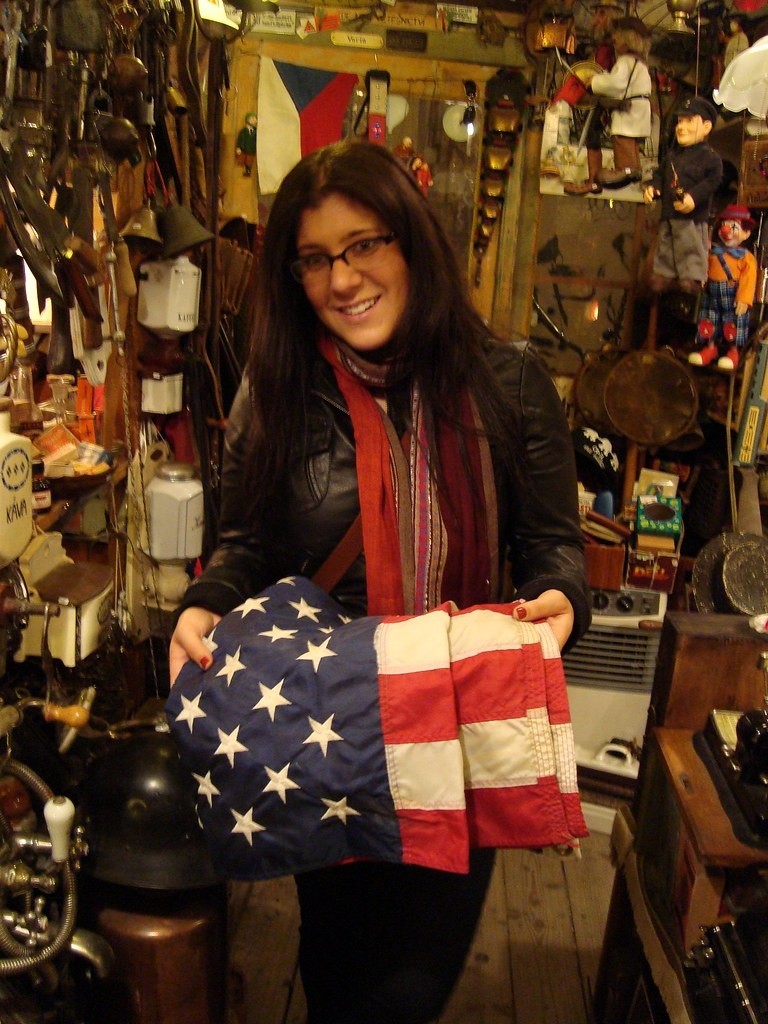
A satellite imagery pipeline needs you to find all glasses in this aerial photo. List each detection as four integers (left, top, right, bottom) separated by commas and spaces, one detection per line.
291, 232, 399, 284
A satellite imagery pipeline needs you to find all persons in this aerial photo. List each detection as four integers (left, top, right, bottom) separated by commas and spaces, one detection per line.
168, 136, 592, 1024
687, 204, 757, 370
643, 96, 723, 294
587, 17, 653, 188
